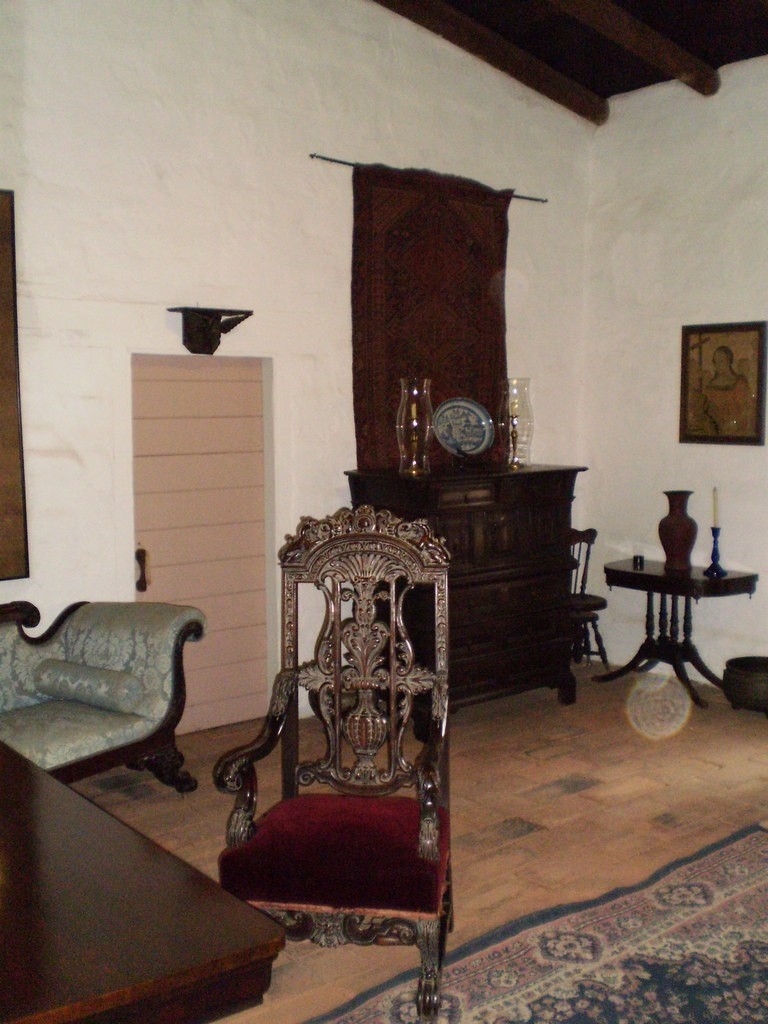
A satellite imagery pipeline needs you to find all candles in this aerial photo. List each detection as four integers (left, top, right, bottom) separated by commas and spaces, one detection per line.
712, 486, 719, 527
512, 402, 517, 417
412, 403, 417, 417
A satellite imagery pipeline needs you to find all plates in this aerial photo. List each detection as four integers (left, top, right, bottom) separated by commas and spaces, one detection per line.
433, 398, 494, 456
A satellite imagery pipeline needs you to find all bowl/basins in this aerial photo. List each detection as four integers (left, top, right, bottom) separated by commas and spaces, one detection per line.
723, 656, 768, 710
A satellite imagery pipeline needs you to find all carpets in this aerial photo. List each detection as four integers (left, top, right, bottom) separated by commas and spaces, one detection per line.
304, 823, 768, 1024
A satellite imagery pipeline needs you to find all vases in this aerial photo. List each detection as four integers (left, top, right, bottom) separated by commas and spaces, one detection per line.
658, 490, 699, 573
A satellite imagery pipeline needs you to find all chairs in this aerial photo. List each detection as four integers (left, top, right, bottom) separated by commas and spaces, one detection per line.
568, 528, 610, 672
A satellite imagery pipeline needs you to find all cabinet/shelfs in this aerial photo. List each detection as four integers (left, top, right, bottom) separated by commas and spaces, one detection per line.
344, 463, 588, 742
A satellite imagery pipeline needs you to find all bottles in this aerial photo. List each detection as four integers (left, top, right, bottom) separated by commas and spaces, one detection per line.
498, 377, 533, 470
395, 376, 432, 475
658, 491, 698, 570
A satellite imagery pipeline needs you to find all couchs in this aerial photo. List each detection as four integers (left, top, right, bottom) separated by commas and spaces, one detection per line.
213, 504, 453, 1024
0, 601, 206, 794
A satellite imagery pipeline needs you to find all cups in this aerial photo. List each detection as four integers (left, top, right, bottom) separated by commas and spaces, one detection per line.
633, 545, 644, 565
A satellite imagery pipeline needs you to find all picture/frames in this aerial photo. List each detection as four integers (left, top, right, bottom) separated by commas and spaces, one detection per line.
679, 320, 768, 447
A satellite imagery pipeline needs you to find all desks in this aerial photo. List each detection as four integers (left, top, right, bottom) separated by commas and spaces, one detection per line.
590, 557, 759, 709
0, 740, 287, 1024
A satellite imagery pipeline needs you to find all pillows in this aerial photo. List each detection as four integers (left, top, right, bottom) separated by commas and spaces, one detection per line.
34, 660, 144, 714
